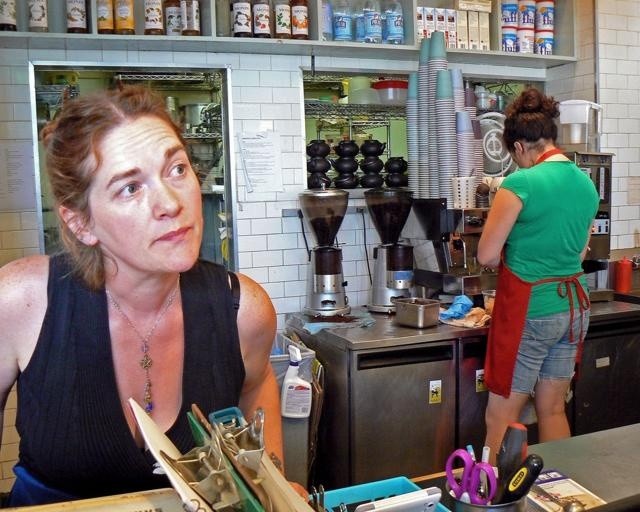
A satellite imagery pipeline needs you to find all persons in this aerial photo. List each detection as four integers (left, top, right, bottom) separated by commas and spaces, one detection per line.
0, 83, 310, 505
476, 87, 602, 465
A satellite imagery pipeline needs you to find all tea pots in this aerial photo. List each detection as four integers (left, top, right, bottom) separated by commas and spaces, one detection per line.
360, 157, 385, 173
306, 140, 332, 158
308, 173, 333, 189
359, 139, 387, 157
385, 156, 408, 174
307, 159, 331, 174
385, 173, 409, 187
336, 140, 360, 157
328, 157, 359, 173
334, 175, 359, 188
359, 174, 385, 188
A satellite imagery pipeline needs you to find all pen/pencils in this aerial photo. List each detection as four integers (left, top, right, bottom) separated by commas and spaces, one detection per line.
481, 446, 491, 491
467, 444, 478, 474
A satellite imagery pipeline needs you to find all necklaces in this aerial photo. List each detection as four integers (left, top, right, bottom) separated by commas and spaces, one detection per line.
101, 281, 180, 417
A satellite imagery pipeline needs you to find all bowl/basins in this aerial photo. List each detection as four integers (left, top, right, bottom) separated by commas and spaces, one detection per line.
371, 81, 408, 104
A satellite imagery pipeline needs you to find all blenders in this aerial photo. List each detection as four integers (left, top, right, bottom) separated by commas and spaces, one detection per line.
363, 187, 416, 318
297, 183, 353, 317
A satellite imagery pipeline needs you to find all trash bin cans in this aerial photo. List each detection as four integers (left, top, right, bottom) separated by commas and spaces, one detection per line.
270, 333, 315, 494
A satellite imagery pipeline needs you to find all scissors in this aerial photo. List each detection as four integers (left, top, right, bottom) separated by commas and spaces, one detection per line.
446, 449, 497, 506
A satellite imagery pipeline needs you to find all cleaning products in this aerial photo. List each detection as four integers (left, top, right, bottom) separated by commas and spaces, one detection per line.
280, 345, 313, 419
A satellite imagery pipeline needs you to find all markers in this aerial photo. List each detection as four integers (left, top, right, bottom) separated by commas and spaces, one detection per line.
496, 422, 543, 504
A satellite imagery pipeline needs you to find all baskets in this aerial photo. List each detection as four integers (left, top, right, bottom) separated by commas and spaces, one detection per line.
307, 476, 452, 512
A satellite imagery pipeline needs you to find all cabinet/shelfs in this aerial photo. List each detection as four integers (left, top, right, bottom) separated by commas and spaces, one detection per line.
2, 1, 579, 70
314, 119, 392, 185
115, 72, 223, 142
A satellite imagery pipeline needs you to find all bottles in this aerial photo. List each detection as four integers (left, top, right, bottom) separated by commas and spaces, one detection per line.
614, 254, 632, 295
144, 1, 164, 35
97, 0, 114, 33
252, 0, 271, 39
216, 0, 230, 36
114, 0, 135, 34
181, 0, 200, 35
322, 0, 405, 44
230, 0, 233, 36
164, 1, 180, 35
291, 0, 309, 39
497, 96, 504, 111
274, 0, 291, 38
66, 0, 88, 33
233, 0, 252, 37
0, 0, 17, 31
268, 0, 274, 39
27, 0, 48, 32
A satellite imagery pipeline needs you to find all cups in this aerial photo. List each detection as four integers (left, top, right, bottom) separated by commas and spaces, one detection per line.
482, 289, 496, 314
406, 31, 489, 209
